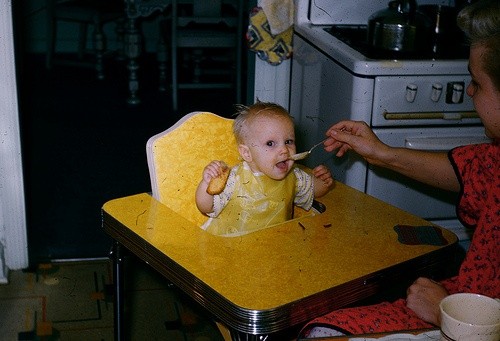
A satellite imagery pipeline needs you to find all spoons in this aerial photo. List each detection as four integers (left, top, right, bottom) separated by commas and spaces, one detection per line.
287, 127, 346, 160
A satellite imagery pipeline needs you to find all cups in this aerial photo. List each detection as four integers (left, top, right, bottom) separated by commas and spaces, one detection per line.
439, 293, 500, 341
416, 4, 461, 61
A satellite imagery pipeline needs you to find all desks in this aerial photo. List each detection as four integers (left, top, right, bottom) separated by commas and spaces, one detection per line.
99, 110, 459, 341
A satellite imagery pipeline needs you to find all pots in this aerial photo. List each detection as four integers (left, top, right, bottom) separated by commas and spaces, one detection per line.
366, 1, 415, 52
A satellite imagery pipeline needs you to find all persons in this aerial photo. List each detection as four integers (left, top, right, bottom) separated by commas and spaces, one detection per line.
196, 99, 335, 237
300, 29, 500, 337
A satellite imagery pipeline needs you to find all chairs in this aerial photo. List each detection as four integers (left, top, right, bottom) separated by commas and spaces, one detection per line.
170, 0, 243, 109
44, 1, 125, 80
144, 111, 322, 237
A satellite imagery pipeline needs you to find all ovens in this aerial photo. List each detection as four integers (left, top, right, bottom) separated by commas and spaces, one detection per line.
290, 35, 493, 240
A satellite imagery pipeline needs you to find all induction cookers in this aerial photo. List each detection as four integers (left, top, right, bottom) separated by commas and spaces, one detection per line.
294, 22, 470, 76
